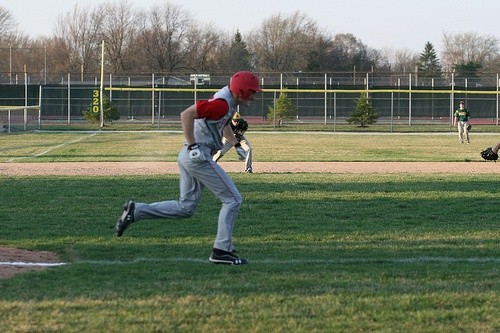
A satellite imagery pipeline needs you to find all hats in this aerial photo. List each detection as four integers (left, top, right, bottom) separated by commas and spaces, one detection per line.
232, 112, 241, 122
460, 101, 465, 105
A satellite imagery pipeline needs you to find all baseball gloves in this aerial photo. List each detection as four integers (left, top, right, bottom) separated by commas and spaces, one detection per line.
480, 147, 499, 161
466, 124, 472, 130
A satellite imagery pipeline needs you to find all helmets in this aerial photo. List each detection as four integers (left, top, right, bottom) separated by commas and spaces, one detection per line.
229, 71, 263, 101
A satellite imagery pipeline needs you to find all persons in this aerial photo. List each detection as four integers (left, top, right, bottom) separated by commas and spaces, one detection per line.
117, 70, 263, 263
454, 101, 470, 144
213, 113, 252, 174
481, 142, 500, 160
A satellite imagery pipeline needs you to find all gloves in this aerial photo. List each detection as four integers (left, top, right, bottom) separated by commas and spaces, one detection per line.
188, 143, 209, 163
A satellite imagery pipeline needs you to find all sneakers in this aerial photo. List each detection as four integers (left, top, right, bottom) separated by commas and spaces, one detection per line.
116, 200, 134, 237
209, 249, 248, 265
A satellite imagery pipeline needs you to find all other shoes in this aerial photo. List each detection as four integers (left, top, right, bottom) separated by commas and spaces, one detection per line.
466, 140, 470, 144
245, 169, 252, 174
